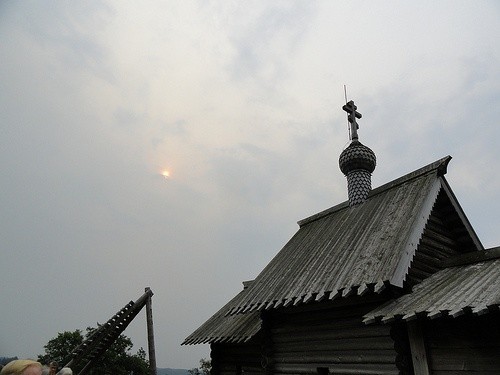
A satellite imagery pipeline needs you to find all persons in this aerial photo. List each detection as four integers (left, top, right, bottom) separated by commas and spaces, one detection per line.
49, 361, 57, 375
1, 360, 43, 375
